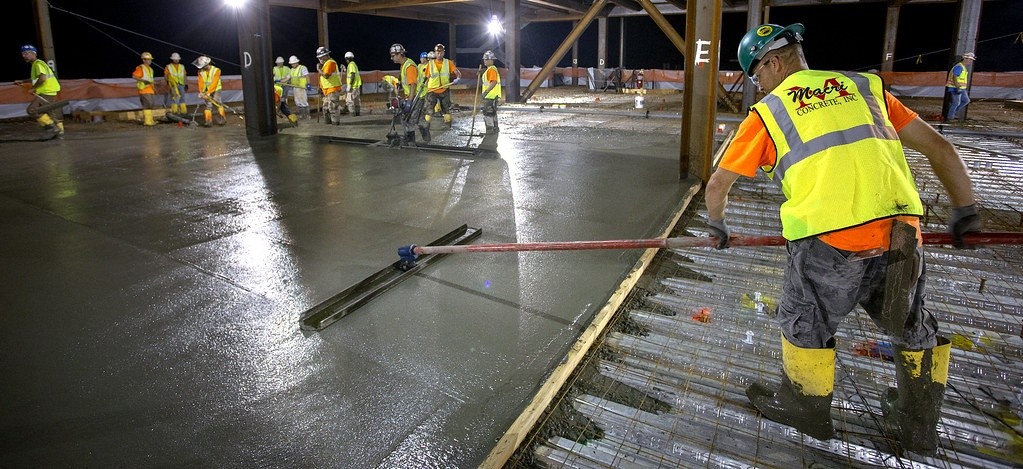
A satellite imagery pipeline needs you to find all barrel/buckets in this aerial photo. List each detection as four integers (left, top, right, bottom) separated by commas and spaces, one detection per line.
635, 96, 645, 108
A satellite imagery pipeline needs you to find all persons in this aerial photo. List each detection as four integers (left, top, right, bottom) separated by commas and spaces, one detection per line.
476, 51, 501, 135
380, 44, 462, 143
14, 44, 64, 141
944, 51, 977, 123
272, 55, 311, 126
164, 53, 189, 114
316, 47, 342, 125
705, 23, 980, 457
340, 51, 362, 117
131, 52, 157, 126
191, 56, 227, 126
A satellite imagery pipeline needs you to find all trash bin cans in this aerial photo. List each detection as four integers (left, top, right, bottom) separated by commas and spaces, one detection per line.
554, 73, 564, 87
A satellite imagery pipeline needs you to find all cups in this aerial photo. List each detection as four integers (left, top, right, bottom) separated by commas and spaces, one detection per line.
719, 124, 725, 130
716, 128, 722, 133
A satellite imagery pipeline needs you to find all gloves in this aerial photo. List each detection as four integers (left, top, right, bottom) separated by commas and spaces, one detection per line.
403, 100, 412, 113
951, 203, 985, 252
707, 217, 731, 251
452, 79, 458, 85
307, 85, 311, 91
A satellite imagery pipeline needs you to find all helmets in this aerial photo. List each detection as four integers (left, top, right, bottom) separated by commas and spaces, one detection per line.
481, 50, 498, 60
274, 44, 445, 66
962, 52, 976, 61
169, 52, 181, 59
737, 22, 805, 74
141, 52, 154, 59
19, 45, 38, 54
191, 56, 211, 69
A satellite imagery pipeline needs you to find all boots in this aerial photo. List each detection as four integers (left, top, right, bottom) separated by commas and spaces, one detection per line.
37, 113, 59, 135
171, 103, 178, 114
142, 110, 156, 125
217, 107, 227, 125
746, 326, 837, 441
202, 109, 214, 127
178, 103, 189, 114
52, 123, 66, 140
879, 334, 954, 451
407, 115, 499, 150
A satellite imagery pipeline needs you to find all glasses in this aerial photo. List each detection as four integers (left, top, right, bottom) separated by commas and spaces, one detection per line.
752, 56, 777, 86
